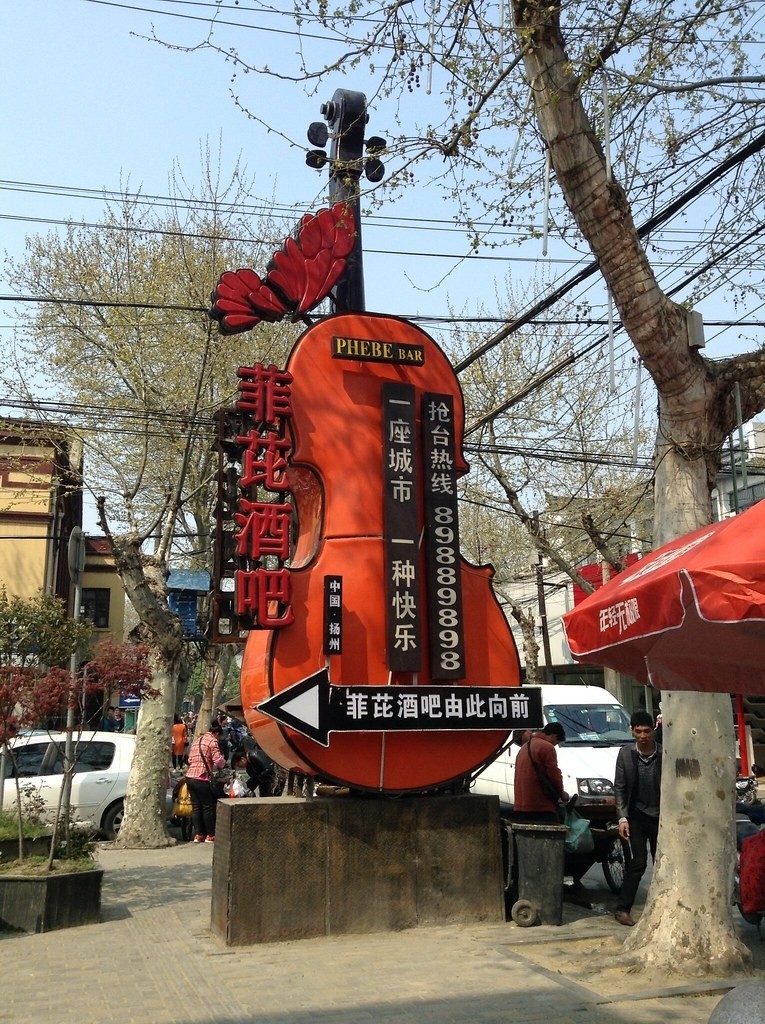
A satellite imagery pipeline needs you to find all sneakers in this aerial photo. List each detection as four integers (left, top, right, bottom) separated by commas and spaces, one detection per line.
204, 835, 215, 843
193, 834, 205, 843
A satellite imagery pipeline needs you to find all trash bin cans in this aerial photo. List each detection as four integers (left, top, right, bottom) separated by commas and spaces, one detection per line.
500, 817, 571, 927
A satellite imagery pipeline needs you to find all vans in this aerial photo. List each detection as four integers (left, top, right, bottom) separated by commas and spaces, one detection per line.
469, 683, 635, 808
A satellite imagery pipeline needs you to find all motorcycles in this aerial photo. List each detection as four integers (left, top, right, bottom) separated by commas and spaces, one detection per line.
735, 767, 765, 899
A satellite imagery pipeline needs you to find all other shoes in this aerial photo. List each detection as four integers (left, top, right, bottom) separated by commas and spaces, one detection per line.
179, 768, 182, 772
173, 769, 177, 773
615, 911, 635, 926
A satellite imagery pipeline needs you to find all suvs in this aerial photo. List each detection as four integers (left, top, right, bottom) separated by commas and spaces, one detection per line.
0, 730, 174, 841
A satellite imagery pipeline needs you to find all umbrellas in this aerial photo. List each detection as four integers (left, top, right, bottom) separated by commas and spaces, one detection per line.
561, 498, 765, 695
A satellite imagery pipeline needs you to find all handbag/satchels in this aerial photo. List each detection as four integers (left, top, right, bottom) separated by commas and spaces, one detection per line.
209, 773, 226, 798
535, 764, 560, 801
562, 805, 594, 855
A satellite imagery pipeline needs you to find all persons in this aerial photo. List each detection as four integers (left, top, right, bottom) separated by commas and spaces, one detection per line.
185, 720, 225, 844
114, 707, 124, 731
171, 714, 186, 772
513, 723, 569, 825
100, 706, 119, 732
231, 747, 287, 797
615, 712, 663, 926
182, 712, 257, 767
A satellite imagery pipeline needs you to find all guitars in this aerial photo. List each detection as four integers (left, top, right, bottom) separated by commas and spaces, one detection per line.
240, 86, 524, 793
